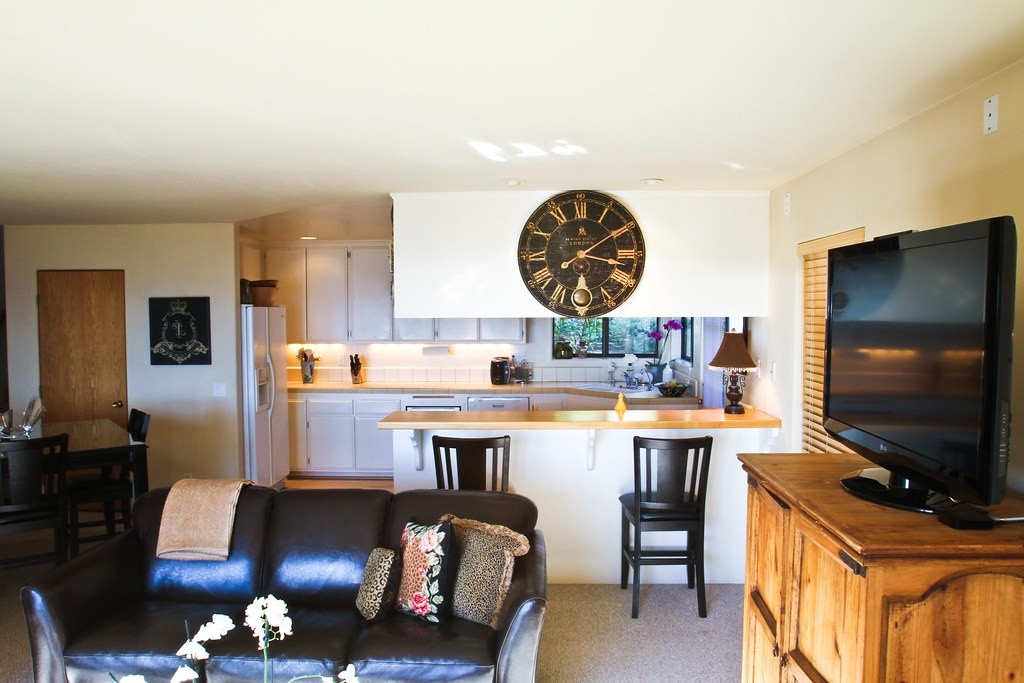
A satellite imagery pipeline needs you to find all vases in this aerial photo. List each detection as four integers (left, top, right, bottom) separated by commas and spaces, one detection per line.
646, 365, 666, 385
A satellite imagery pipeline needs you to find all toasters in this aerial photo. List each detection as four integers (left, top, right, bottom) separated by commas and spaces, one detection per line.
490, 356, 511, 384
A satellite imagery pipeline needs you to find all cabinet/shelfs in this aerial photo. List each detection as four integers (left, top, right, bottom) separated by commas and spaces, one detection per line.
530, 394, 567, 412
286, 392, 402, 479
263, 240, 526, 345
737, 453, 1024, 683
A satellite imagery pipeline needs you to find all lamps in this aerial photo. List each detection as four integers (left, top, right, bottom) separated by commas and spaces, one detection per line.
708, 332, 757, 415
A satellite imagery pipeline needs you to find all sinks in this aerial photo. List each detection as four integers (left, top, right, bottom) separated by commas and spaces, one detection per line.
613, 389, 645, 393
578, 385, 618, 392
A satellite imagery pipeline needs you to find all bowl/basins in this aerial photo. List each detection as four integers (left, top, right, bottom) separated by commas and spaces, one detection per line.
654, 382, 689, 396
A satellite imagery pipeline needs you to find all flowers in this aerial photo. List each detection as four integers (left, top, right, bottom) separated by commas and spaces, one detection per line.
109, 593, 361, 683
644, 320, 683, 367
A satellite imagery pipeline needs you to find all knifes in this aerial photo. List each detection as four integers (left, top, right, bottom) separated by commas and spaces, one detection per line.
350, 354, 361, 376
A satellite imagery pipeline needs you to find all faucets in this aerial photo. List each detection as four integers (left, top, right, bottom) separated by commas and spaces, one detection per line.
620, 372, 631, 389
641, 368, 651, 391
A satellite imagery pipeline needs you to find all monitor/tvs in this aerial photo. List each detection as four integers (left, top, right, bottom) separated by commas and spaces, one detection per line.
821, 216, 1017, 513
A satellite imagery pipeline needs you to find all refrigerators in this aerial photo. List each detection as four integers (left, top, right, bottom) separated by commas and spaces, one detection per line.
240, 302, 291, 493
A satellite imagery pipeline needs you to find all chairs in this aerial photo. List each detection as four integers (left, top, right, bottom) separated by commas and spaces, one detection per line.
0, 408, 151, 572
432, 435, 511, 493
618, 436, 713, 619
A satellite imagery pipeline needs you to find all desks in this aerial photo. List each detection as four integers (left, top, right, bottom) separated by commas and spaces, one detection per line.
0, 418, 149, 566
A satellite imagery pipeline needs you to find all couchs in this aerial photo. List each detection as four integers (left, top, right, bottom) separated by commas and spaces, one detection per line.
20, 478, 548, 683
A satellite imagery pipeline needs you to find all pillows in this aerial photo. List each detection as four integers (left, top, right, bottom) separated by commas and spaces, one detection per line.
357, 514, 531, 632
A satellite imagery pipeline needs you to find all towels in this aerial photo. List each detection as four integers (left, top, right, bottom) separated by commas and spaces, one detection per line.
155, 479, 257, 561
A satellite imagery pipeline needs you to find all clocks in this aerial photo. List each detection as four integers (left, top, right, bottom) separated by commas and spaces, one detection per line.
517, 190, 645, 319
